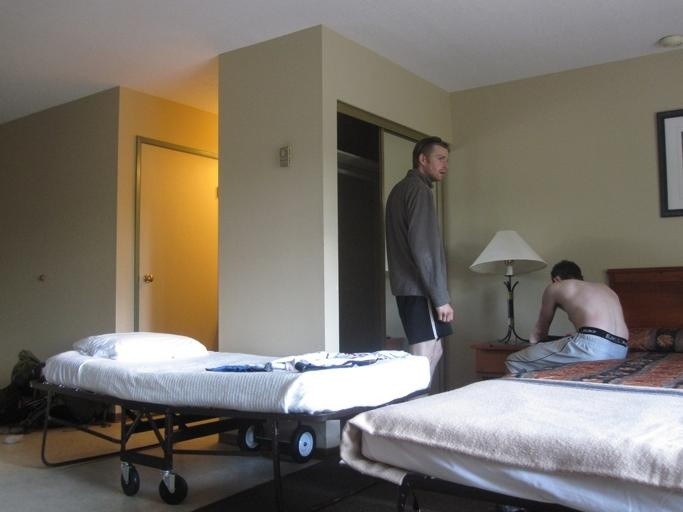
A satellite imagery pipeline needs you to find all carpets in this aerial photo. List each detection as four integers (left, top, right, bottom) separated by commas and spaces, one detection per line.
184, 456, 498, 510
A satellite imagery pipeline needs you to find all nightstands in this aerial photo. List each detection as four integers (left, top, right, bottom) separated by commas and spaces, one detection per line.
471, 342, 530, 382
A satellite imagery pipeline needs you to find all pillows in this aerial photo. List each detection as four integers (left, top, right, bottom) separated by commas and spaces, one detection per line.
626, 326, 683, 354
72, 331, 207, 358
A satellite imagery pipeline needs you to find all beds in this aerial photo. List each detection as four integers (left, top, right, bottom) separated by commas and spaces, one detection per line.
27, 350, 429, 505
338, 266, 683, 510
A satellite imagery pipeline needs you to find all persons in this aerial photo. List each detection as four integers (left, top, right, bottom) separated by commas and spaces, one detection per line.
502, 260, 630, 374
386, 135, 456, 400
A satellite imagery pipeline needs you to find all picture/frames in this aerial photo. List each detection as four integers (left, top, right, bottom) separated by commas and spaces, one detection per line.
656, 108, 683, 217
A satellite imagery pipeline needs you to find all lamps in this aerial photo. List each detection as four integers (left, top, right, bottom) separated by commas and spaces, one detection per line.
468, 230, 547, 346
